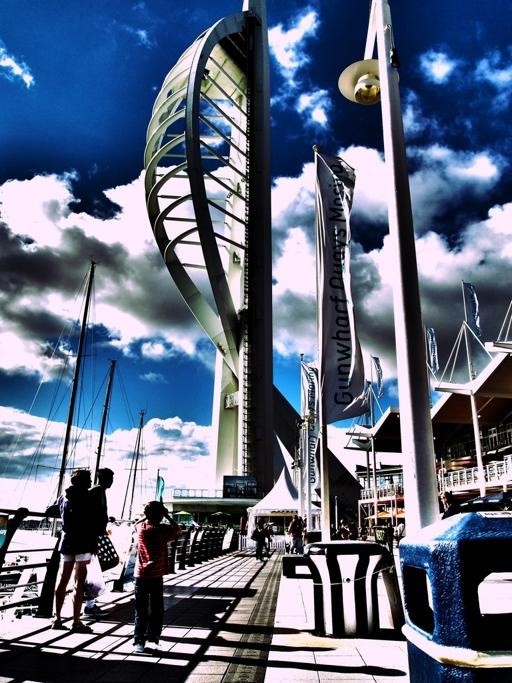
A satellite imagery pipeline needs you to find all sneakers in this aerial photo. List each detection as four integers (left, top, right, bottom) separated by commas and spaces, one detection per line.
135, 644, 145, 653
70, 623, 94, 634
51, 619, 61, 630
144, 641, 163, 654
84, 605, 109, 616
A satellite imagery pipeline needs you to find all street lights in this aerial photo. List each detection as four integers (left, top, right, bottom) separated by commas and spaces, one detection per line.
339, 0, 440, 533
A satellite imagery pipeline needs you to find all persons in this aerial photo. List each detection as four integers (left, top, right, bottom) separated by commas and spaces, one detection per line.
132, 501, 182, 653
396, 523, 405, 548
83, 467, 115, 617
250, 514, 307, 562
46, 468, 102, 634
330, 518, 358, 540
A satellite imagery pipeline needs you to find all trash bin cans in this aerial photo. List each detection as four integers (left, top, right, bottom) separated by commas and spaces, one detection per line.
399, 510, 512, 683
